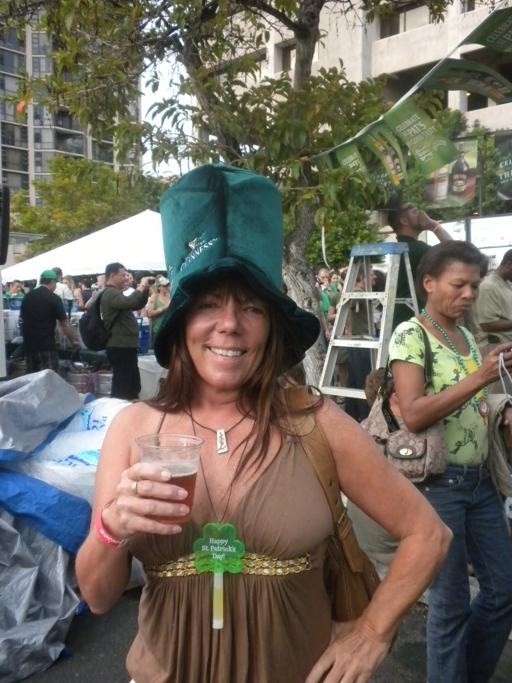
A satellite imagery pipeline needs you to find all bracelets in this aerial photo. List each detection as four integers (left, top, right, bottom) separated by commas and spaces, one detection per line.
433, 223, 440, 232
93, 496, 132, 553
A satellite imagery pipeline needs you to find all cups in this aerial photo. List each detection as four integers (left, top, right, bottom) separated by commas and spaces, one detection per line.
136, 433, 204, 525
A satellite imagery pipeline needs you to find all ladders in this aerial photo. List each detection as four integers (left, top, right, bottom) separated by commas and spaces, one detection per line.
315, 241, 420, 426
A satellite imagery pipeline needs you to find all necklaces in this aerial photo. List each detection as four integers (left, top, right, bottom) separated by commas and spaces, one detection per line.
422, 309, 492, 421
172, 387, 262, 452
179, 387, 264, 631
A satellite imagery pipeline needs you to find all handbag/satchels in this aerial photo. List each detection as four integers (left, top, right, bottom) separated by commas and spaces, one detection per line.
283, 382, 402, 657
358, 320, 450, 487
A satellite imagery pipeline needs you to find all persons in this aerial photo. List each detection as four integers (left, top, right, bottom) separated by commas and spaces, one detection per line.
72, 164, 449, 682
317, 259, 393, 409
1, 264, 170, 400
450, 148, 470, 196
390, 242, 511, 682
475, 249, 512, 365
383, 201, 454, 322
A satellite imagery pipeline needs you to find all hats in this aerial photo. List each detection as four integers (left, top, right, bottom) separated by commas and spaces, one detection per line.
155, 275, 171, 287
151, 163, 323, 371
39, 269, 60, 283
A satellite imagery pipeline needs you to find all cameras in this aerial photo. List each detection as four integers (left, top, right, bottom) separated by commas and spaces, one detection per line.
146, 278, 156, 286
